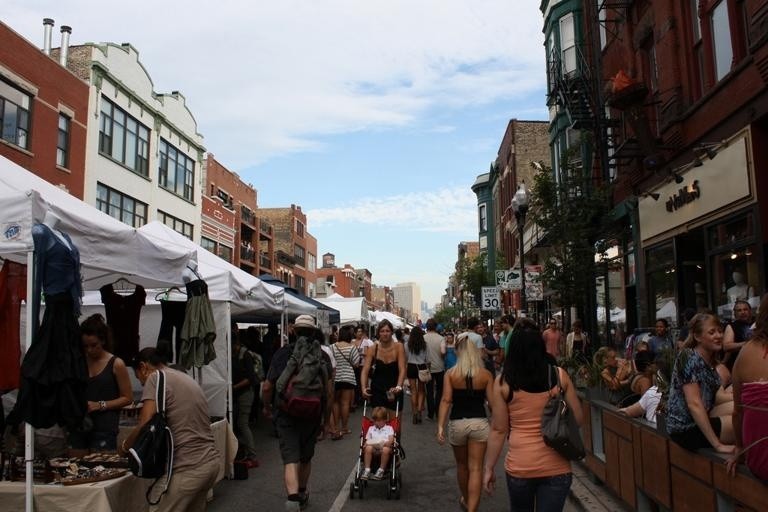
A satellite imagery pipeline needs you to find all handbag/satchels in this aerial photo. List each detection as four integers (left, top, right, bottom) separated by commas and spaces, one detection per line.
121, 439, 130, 453
98, 401, 107, 410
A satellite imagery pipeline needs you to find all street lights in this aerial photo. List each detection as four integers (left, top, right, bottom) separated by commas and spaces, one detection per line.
541, 395, 585, 460
418, 368, 432, 382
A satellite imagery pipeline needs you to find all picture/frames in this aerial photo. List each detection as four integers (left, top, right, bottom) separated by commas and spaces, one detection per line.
0, 419, 239, 512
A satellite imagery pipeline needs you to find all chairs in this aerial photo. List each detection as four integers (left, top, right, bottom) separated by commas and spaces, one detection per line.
238, 458, 259, 468
373, 469, 384, 480
286, 488, 309, 512
360, 471, 371, 481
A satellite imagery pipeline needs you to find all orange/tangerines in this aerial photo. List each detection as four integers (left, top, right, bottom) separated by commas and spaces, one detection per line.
349, 389, 401, 501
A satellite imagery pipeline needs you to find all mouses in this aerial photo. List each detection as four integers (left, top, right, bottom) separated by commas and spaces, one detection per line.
112, 274, 137, 293
155, 286, 187, 301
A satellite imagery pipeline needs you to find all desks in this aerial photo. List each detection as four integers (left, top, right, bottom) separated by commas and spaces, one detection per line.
448, 301, 453, 327
452, 296, 457, 328
458, 281, 465, 330
511, 179, 530, 317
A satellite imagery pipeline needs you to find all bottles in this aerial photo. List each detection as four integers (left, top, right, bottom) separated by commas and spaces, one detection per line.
289, 315, 321, 335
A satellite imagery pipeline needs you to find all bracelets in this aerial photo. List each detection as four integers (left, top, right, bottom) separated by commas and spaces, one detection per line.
98, 401, 107, 410
121, 439, 130, 453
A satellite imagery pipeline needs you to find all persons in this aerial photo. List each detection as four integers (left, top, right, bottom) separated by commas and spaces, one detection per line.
176, 259, 216, 370
117, 347, 221, 511
725, 270, 755, 302
240, 239, 253, 259
73, 314, 133, 451
724, 292, 768, 487
225, 298, 766, 512
5, 211, 86, 429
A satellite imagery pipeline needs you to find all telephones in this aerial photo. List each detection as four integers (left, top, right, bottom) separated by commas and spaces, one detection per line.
481, 285, 501, 311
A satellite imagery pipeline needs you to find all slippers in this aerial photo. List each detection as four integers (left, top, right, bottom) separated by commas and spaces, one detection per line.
129, 369, 174, 505
274, 335, 328, 418
239, 347, 264, 380
